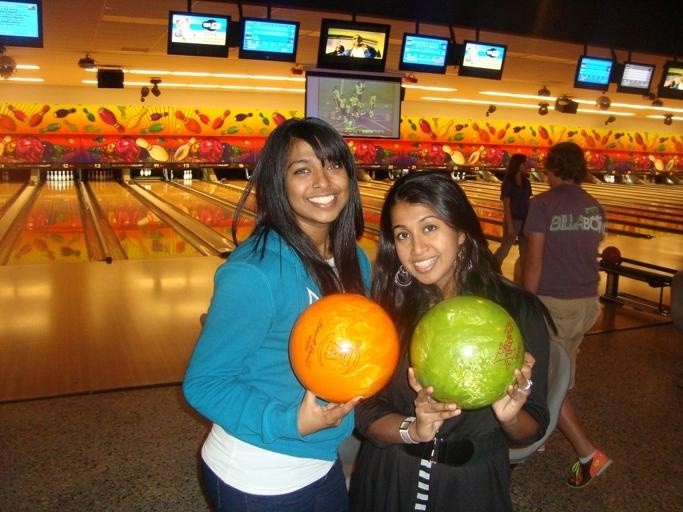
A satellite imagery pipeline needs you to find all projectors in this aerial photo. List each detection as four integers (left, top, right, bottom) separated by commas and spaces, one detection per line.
554, 97, 578, 113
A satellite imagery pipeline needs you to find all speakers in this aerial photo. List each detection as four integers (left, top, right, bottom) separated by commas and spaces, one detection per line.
97, 68, 124, 88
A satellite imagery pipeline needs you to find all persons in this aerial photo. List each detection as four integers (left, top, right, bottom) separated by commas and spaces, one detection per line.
326, 45, 344, 57
516, 142, 612, 488
491, 153, 533, 273
183, 116, 373, 511
348, 169, 559, 511
342, 37, 381, 60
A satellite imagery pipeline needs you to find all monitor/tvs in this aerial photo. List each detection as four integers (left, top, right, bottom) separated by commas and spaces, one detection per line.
0, 0, 44, 48
317, 17, 391, 73
238, 16, 300, 61
657, 60, 683, 100
616, 61, 655, 95
573, 54, 615, 91
398, 32, 451, 75
167, 10, 231, 58
458, 40, 507, 80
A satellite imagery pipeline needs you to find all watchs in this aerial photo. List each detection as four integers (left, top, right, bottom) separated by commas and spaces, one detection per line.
398, 414, 420, 445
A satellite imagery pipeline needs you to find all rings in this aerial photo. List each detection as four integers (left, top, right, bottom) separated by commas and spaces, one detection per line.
520, 378, 532, 389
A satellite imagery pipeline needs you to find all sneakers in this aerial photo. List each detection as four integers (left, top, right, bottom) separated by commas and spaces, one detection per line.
563, 449, 613, 490
